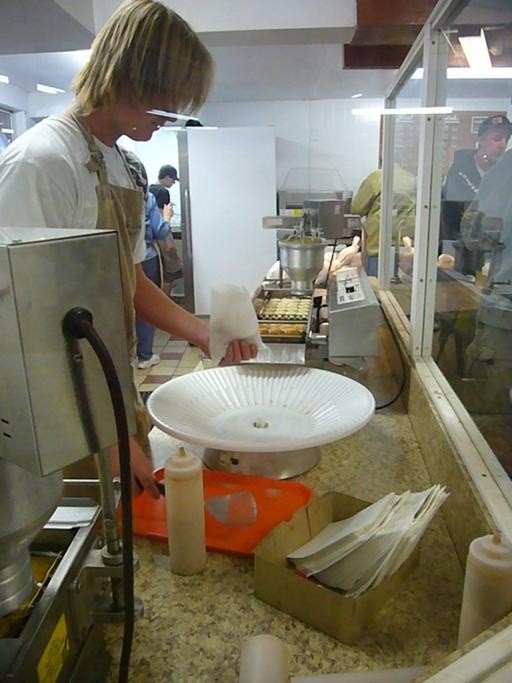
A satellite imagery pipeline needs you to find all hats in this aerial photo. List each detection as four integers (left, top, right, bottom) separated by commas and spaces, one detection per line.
160, 165, 180, 181
478, 115, 512, 136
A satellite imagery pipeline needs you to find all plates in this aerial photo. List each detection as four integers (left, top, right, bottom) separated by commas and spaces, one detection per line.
146, 364, 376, 453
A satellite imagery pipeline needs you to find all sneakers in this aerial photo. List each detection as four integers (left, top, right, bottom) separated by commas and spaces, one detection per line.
137, 354, 161, 369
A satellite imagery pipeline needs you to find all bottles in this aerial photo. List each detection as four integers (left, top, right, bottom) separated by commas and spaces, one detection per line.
163, 446, 208, 576
456, 528, 512, 649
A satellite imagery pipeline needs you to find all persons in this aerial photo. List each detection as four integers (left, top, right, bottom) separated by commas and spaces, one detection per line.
136, 186, 174, 369
149, 164, 182, 297
459, 147, 512, 472
0, 0, 258, 499
446, 115, 512, 375
349, 146, 418, 280
